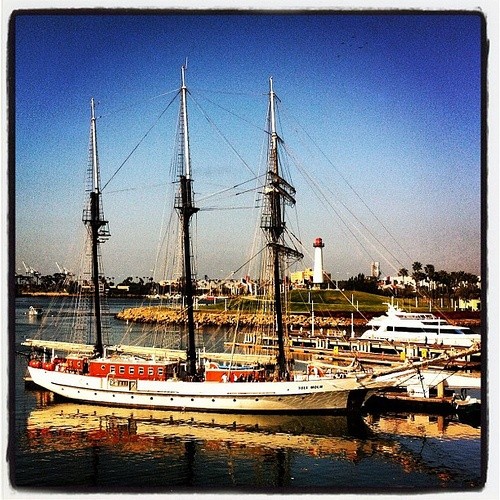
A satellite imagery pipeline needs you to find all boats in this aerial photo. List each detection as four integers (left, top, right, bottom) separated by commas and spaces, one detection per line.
224, 336, 480, 390
356, 291, 481, 354
28, 306, 42, 319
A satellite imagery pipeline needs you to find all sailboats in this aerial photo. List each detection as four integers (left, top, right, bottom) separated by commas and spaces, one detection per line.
24, 405, 405, 495
28, 66, 397, 414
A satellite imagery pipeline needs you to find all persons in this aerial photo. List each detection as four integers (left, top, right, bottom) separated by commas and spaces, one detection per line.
174, 369, 291, 384
